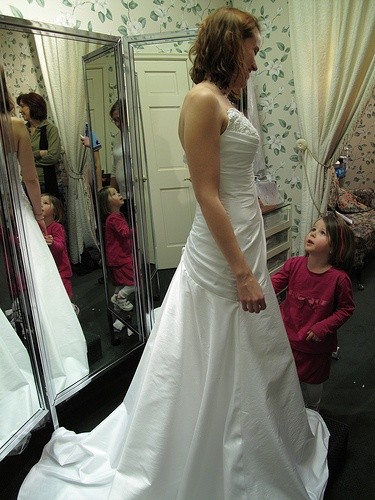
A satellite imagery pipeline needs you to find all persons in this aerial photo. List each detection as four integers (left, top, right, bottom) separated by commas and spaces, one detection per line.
14, 6, 332, 500
264, 208, 355, 422
1, 62, 89, 399
37, 192, 83, 316
80, 126, 103, 196
12, 92, 67, 218
97, 186, 139, 313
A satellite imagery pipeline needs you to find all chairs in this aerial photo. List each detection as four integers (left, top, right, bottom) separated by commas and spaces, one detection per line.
328, 166, 375, 291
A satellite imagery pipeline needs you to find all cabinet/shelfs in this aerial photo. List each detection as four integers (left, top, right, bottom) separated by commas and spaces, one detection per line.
262, 202, 291, 278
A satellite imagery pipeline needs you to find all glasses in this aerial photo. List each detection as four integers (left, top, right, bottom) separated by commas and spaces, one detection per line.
111, 118, 120, 123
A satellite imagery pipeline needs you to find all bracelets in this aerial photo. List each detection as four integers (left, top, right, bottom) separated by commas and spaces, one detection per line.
36, 217, 44, 222
34, 213, 43, 216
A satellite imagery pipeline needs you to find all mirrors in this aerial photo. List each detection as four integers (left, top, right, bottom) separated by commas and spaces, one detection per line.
0, 15, 201, 490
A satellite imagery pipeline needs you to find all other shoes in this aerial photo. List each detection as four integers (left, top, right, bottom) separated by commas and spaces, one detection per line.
111, 294, 133, 311
72, 304, 80, 315
